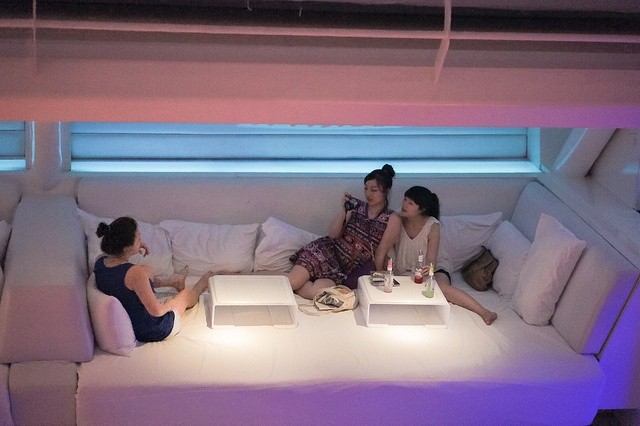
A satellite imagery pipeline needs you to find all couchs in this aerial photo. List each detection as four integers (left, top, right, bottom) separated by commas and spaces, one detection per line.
1, 178, 637, 426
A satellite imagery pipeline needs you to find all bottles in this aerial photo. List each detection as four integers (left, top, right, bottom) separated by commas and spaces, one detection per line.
426, 265, 435, 298
384, 260, 394, 292
414, 251, 424, 283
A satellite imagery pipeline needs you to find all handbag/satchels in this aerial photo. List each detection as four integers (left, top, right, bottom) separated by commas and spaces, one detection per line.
298, 285, 359, 316
461, 245, 499, 291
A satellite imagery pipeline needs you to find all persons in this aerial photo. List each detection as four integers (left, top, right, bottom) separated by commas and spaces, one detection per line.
92, 217, 213, 343
287, 164, 402, 300
395, 186, 497, 326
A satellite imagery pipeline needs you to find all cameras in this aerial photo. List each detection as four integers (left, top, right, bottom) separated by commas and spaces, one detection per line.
344, 198, 359, 211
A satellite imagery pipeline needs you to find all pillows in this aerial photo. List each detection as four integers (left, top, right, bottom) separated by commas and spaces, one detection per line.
511, 213, 586, 326
484, 219, 531, 302
0, 220, 13, 260
87, 272, 138, 356
254, 215, 326, 274
160, 219, 261, 274
436, 211, 504, 274
76, 207, 173, 276
